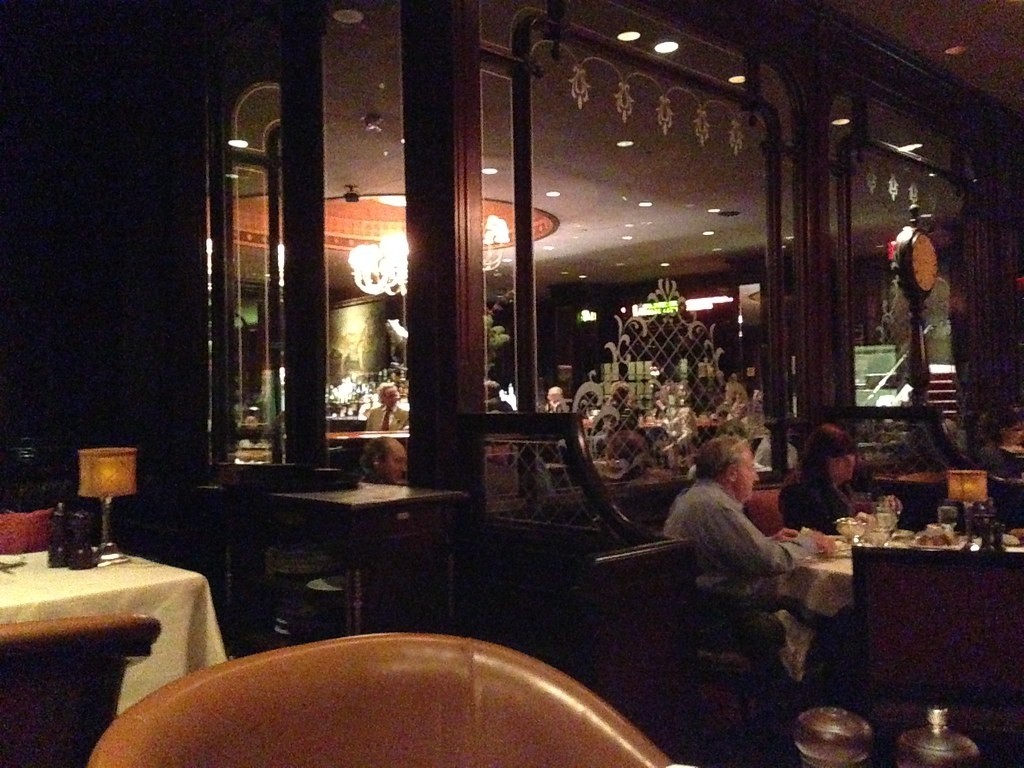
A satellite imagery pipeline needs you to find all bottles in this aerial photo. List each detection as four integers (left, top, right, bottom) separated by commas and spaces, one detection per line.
978, 518, 1005, 552
48, 502, 70, 568
70, 503, 93, 569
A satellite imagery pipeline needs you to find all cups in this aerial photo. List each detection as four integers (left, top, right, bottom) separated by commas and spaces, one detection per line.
836, 507, 896, 548
938, 505, 957, 532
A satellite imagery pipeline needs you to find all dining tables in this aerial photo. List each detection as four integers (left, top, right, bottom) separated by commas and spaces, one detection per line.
852, 541, 1024, 692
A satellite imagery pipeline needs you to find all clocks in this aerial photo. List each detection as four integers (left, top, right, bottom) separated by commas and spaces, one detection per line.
877, 204, 940, 403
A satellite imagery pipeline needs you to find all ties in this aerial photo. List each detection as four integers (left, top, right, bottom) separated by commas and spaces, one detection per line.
382, 409, 391, 431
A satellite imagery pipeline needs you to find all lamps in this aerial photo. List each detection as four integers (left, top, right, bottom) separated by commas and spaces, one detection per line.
76, 447, 137, 567
348, 214, 511, 297
946, 470, 990, 537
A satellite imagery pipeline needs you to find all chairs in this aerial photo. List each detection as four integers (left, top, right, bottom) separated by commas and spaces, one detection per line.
748, 489, 787, 537
85, 633, 676, 768
649, 533, 754, 724
0, 615, 163, 768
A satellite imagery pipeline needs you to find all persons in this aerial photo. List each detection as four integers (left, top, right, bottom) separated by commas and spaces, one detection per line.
898, 405, 1024, 506
664, 374, 833, 701
546, 387, 570, 413
651, 383, 697, 454
366, 382, 410, 431
775, 423, 902, 535
360, 437, 407, 484
487, 381, 511, 410
587, 382, 640, 453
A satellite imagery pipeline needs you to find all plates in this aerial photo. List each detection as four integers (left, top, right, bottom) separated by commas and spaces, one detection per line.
891, 529, 915, 538
911, 536, 968, 550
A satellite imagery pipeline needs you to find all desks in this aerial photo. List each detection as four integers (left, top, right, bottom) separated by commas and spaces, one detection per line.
195, 485, 474, 654
0, 547, 229, 719
